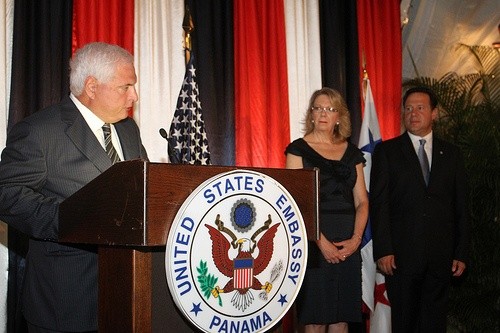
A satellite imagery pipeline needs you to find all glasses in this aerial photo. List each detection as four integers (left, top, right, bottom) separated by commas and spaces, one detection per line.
312, 106, 338, 114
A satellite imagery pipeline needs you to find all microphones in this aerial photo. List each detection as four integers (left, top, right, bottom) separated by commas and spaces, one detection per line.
159, 128, 180, 163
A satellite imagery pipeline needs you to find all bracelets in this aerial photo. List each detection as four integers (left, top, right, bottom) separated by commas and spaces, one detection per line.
354, 233, 362, 240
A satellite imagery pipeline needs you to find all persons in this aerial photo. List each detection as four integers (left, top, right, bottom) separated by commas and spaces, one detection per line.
369, 86, 472, 333
282, 88, 369, 333
0, 42, 148, 333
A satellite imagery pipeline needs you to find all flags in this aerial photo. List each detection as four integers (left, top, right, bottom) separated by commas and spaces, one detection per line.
167, 53, 211, 165
356, 77, 392, 333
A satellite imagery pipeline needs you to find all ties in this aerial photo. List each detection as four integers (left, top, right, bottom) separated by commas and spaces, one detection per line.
101, 123, 120, 164
419, 139, 430, 186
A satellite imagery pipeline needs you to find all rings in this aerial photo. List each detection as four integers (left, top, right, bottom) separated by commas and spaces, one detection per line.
343, 255, 345, 257
